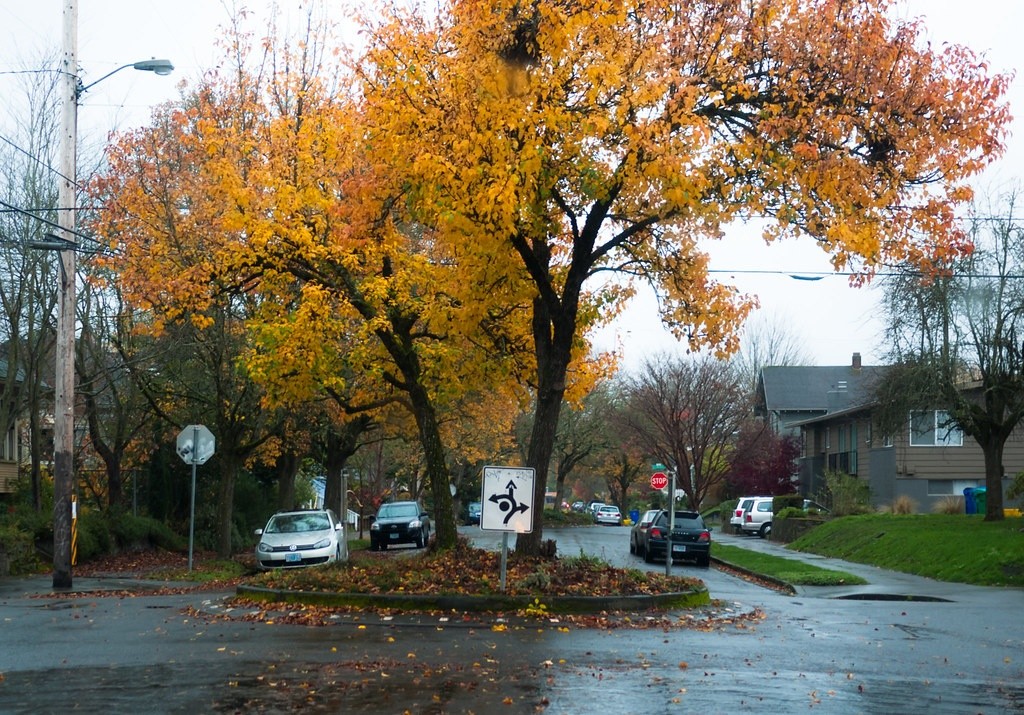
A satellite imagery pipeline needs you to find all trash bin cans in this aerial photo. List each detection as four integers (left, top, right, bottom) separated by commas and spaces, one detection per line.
974, 487, 986, 514
963, 487, 976, 514
630, 510, 639, 524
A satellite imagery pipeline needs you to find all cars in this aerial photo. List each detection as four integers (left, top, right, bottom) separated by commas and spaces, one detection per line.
466, 501, 482, 525
253, 509, 348, 574
572, 501, 606, 516
592, 504, 622, 527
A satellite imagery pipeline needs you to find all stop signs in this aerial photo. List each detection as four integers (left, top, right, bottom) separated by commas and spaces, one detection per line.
650, 472, 669, 489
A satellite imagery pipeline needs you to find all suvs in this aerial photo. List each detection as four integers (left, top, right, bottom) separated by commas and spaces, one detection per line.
729, 494, 831, 540
369, 498, 431, 552
642, 509, 714, 568
630, 510, 661, 556
